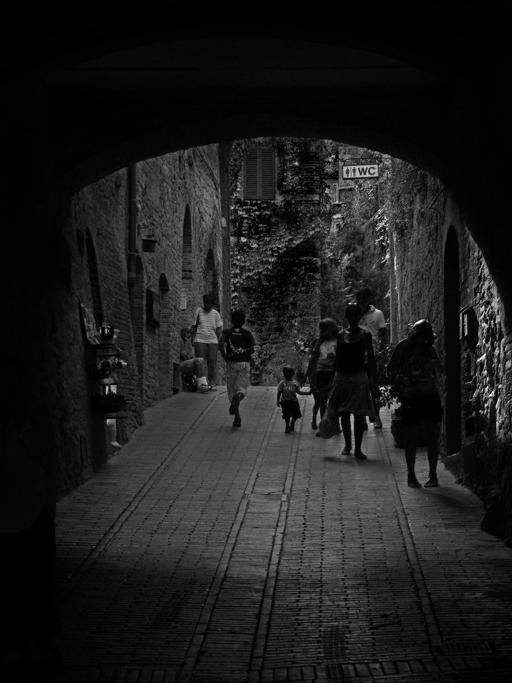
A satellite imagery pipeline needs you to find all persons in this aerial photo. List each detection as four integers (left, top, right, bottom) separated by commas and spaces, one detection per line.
276, 365, 312, 433
385, 319, 447, 488
355, 287, 387, 429
218, 309, 256, 428
190, 294, 224, 393
330, 302, 377, 460
300, 318, 339, 430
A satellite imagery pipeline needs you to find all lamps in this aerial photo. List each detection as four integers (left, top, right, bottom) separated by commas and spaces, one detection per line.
138, 222, 158, 252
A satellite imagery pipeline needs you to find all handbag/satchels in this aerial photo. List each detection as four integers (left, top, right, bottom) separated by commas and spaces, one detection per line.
319, 409, 339, 438
